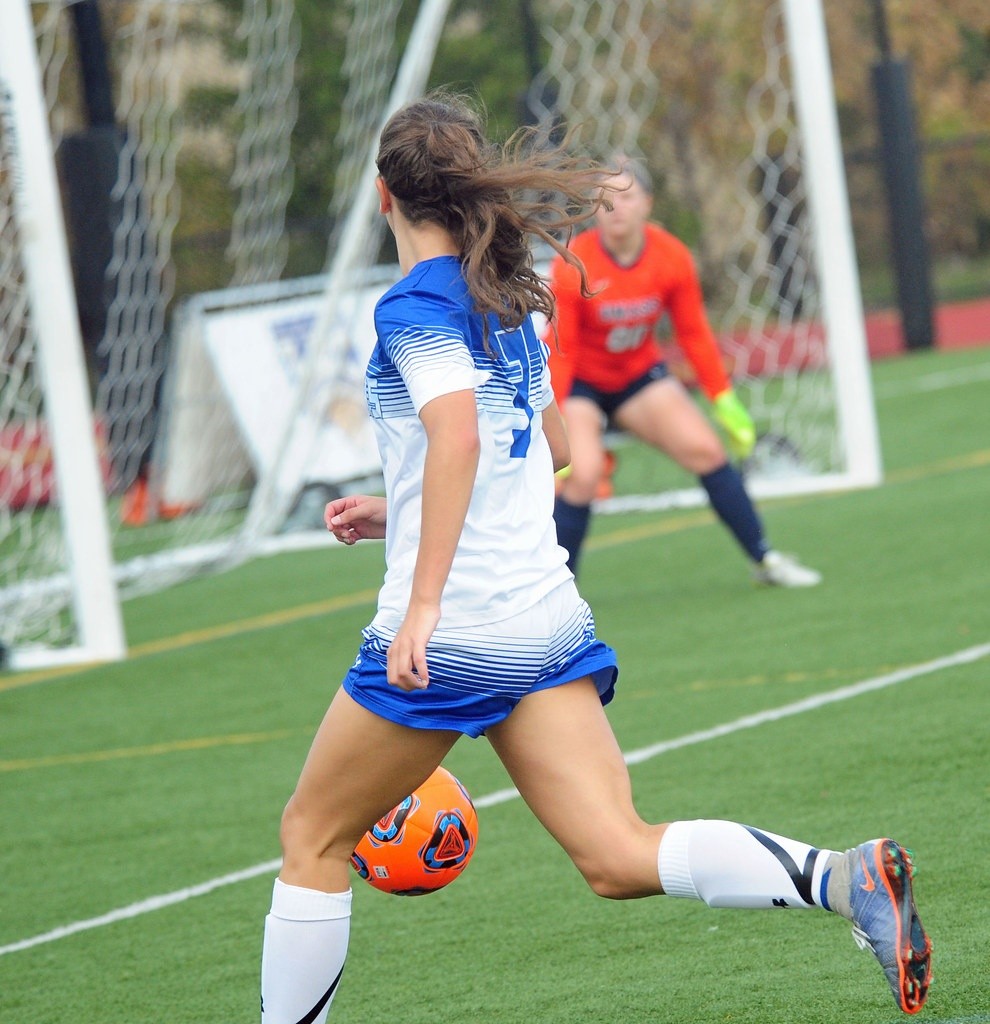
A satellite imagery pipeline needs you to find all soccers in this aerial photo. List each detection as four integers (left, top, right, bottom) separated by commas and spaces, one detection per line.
350, 765, 480, 898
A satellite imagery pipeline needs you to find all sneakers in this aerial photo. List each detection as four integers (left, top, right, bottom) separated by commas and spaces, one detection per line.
758, 550, 822, 588
850, 838, 934, 1014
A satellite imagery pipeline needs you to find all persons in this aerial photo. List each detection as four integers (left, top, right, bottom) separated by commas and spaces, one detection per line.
544, 149, 824, 588
261, 81, 932, 1024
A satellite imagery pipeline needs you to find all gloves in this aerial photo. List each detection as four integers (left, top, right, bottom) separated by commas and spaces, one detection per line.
714, 388, 756, 459
